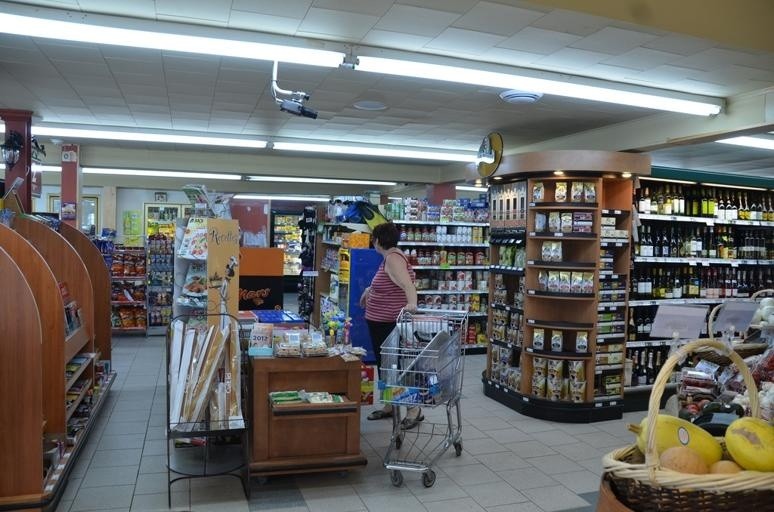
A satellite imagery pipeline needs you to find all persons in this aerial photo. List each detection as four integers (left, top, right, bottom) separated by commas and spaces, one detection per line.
359, 222, 424, 431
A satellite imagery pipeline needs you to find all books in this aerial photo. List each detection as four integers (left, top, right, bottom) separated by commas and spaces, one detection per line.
66, 352, 96, 409
58, 280, 79, 338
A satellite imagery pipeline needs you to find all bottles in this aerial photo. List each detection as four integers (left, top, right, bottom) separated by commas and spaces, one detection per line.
403, 248, 417, 265
626, 184, 774, 386
398, 225, 437, 242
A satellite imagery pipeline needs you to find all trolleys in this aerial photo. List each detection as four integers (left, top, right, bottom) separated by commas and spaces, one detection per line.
380, 306, 468, 487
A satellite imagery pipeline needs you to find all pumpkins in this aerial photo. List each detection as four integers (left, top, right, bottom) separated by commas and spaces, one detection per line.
627, 414, 774, 476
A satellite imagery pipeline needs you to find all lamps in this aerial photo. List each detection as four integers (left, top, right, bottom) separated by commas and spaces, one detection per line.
0, 120, 268, 150
717, 134, 774, 151
498, 88, 543, 105
0, 164, 246, 181
234, 193, 333, 203
267, 136, 481, 164
348, 41, 729, 119
245, 175, 398, 187
0, 0, 350, 69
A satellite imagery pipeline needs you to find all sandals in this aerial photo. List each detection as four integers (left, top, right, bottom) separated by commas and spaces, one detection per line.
367, 406, 394, 421
402, 406, 424, 429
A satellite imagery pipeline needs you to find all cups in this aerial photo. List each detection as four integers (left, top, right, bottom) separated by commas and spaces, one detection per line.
414, 250, 490, 312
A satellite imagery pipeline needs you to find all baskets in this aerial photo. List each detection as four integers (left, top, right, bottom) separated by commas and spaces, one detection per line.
691, 289, 774, 372
605, 340, 774, 512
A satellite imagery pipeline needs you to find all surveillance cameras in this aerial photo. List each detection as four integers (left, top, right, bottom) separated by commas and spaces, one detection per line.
280, 91, 319, 119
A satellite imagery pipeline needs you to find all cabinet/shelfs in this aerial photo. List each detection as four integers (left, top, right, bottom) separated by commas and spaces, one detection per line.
313, 203, 489, 355
241, 350, 369, 477
89, 233, 148, 336
623, 175, 774, 413
481, 168, 633, 425
169, 215, 243, 445
0, 175, 118, 512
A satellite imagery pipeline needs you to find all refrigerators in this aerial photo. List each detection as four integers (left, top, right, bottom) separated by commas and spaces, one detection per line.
271, 208, 316, 292
